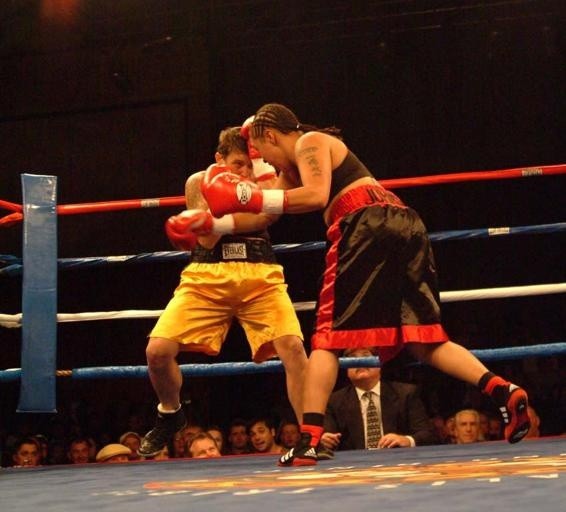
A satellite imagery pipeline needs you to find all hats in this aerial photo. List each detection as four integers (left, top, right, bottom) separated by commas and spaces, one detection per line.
95, 444, 131, 463
120, 432, 140, 447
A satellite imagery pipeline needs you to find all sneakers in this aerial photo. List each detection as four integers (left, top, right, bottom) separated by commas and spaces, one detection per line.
277, 446, 317, 467
135, 404, 187, 459
314, 443, 334, 460
496, 382, 530, 444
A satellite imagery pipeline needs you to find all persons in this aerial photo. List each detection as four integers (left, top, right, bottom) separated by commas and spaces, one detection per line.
163, 103, 533, 467
490, 359, 566, 436
172, 417, 299, 459
136, 126, 338, 462
320, 349, 505, 452
2, 399, 168, 467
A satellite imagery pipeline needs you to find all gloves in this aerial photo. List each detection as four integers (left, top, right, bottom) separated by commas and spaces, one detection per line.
203, 171, 263, 218
240, 114, 262, 158
200, 164, 241, 200
164, 209, 211, 251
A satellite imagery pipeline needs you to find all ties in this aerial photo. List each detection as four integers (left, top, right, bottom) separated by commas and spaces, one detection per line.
363, 391, 381, 449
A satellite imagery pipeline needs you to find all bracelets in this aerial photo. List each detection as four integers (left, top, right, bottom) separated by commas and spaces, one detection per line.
283, 188, 289, 215
231, 214, 237, 235
